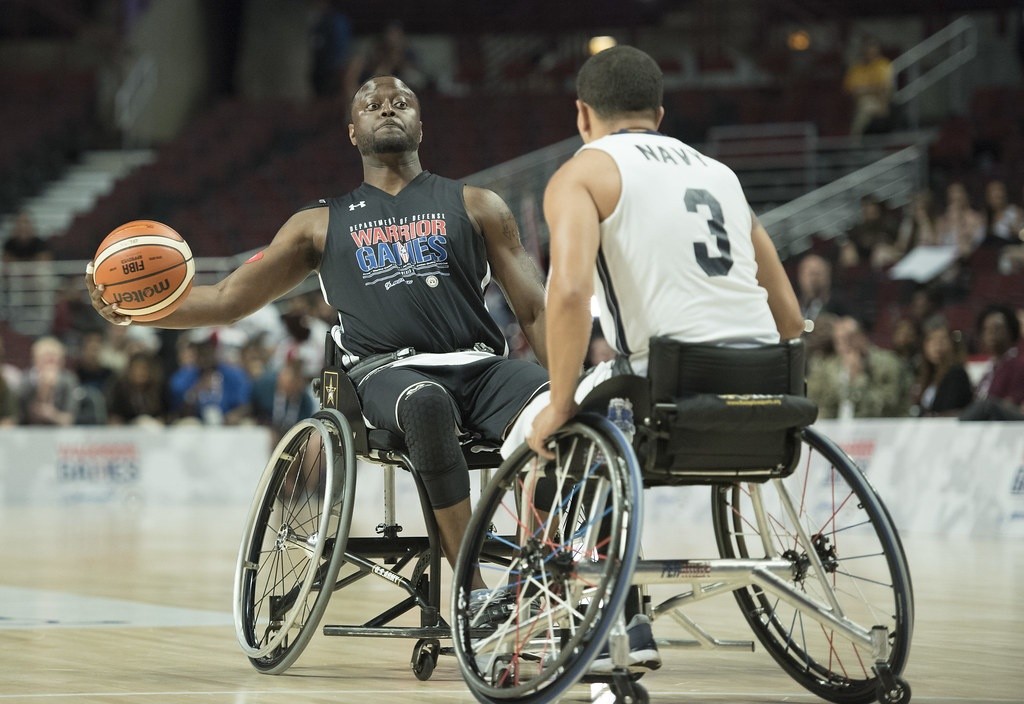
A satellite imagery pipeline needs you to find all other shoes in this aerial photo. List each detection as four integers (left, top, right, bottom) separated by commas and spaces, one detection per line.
461, 589, 515, 680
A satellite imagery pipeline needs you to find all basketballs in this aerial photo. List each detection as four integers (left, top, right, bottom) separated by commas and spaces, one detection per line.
92, 218, 198, 324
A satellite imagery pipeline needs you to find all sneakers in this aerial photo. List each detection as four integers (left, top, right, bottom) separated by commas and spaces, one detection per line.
588, 614, 662, 672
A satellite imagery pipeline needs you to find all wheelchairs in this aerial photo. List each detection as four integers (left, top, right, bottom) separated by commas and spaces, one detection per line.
449, 334, 915, 704
233, 329, 533, 679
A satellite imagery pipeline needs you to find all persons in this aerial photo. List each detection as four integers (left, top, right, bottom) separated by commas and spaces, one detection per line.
0, 266, 343, 501
796, 179, 1024, 423
86, 76, 584, 647
499, 48, 803, 669
846, 36, 900, 148
